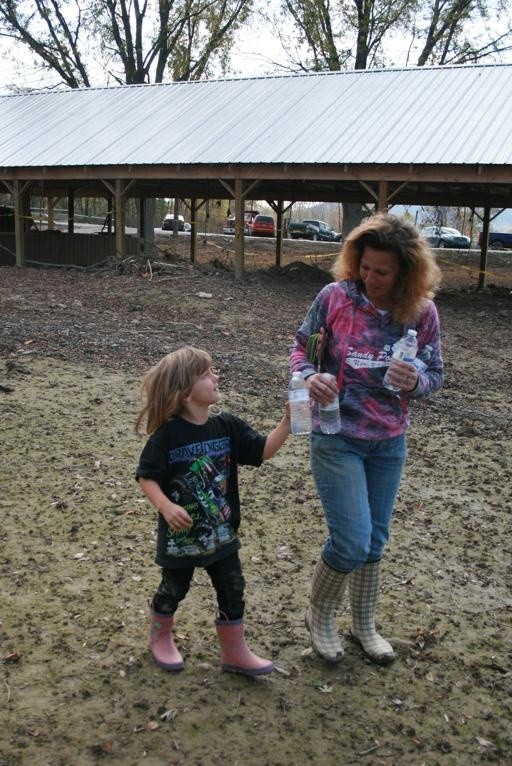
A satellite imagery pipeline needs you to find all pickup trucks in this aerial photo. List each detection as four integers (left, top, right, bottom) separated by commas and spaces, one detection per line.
476, 231, 511, 250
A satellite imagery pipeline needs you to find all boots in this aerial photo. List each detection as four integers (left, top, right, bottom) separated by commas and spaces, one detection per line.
344, 558, 398, 664
303, 550, 350, 664
147, 595, 185, 671
214, 611, 274, 676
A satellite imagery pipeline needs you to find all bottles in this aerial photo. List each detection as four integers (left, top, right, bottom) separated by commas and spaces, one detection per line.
383, 329, 434, 394
286, 371, 312, 437
318, 378, 342, 435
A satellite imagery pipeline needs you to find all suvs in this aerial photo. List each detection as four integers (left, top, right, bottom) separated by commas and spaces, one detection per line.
223, 205, 342, 242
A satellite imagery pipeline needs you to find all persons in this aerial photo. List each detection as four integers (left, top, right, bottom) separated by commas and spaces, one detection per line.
291, 212, 445, 665
133, 346, 315, 676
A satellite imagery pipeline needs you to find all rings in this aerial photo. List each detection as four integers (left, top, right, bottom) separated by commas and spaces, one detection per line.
404, 379, 411, 384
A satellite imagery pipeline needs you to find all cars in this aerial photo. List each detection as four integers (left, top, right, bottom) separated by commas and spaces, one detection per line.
161, 214, 186, 232
420, 226, 473, 251
